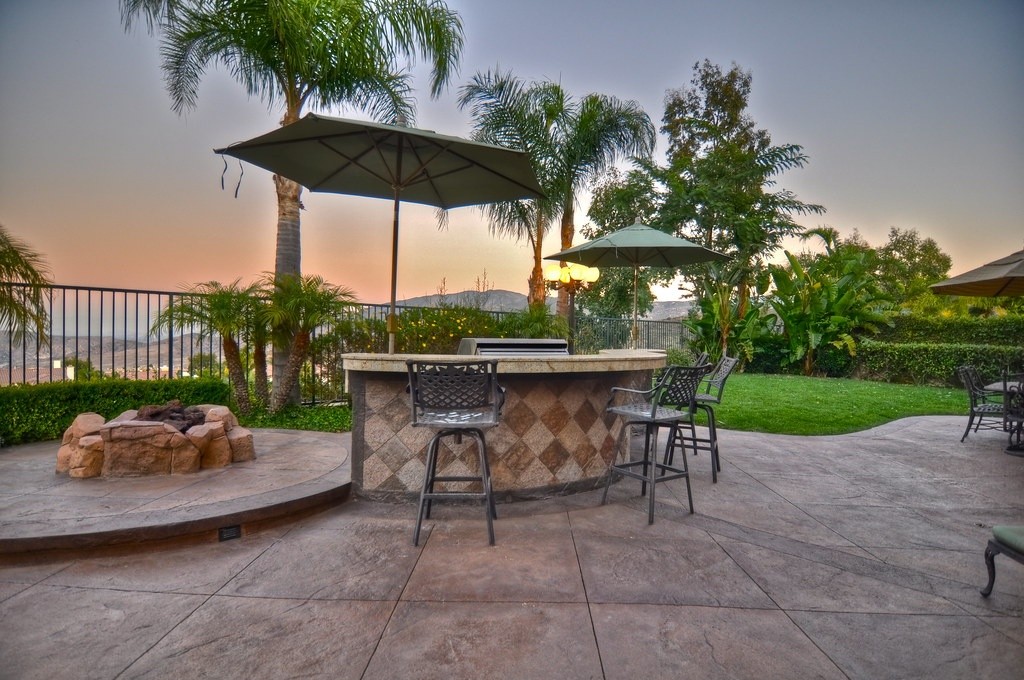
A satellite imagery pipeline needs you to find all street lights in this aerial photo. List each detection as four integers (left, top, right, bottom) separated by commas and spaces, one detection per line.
546, 261, 600, 356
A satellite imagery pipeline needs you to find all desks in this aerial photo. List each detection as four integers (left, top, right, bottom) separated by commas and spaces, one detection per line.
983, 381, 1024, 433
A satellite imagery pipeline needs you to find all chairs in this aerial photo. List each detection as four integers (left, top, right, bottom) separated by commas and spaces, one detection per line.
600, 363, 715, 524
967, 366, 1013, 432
653, 357, 739, 485
404, 356, 503, 549
958, 368, 1015, 444
979, 526, 1024, 598
1001, 365, 1024, 457
683, 352, 710, 367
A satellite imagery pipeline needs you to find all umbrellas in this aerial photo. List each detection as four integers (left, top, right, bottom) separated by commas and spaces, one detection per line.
927, 249, 1024, 297
543, 218, 738, 349
214, 112, 551, 355
